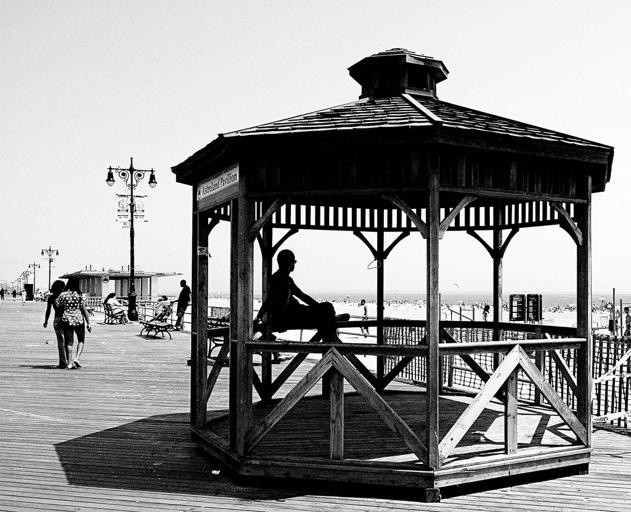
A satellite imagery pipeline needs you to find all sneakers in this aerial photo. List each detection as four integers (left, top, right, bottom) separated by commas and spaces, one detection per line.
74, 359, 82, 368
335, 314, 350, 321
67, 364, 73, 369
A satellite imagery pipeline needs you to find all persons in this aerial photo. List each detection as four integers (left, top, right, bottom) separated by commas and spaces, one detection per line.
360, 300, 370, 334
482, 304, 490, 321
0, 277, 191, 369
254, 250, 350, 342
607, 307, 621, 336
623, 307, 631, 335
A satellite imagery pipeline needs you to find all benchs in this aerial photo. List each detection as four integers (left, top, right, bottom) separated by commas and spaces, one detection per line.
139, 320, 173, 339
207, 317, 233, 359
102, 302, 125, 325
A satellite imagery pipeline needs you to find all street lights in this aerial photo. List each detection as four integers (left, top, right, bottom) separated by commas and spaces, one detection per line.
28, 262, 40, 293
106, 156, 157, 321
10, 270, 31, 295
41, 246, 59, 291
